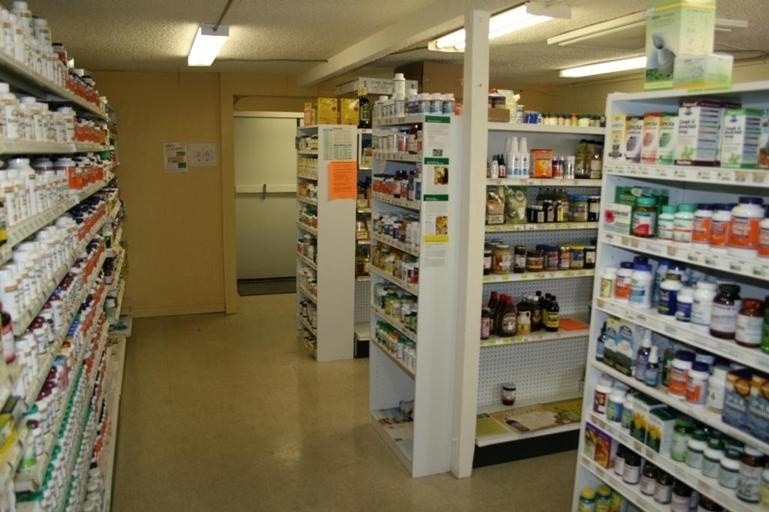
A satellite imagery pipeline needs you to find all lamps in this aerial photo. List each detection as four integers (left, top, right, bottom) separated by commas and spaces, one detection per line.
561, 51, 645, 78
188, 25, 228, 66
428, 1, 572, 54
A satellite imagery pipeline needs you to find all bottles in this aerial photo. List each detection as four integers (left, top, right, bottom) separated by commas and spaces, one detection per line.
357, 87, 371, 129
489, 136, 576, 179
502, 384, 517, 406
526, 187, 588, 222
381, 285, 417, 334
371, 126, 422, 154
371, 92, 455, 118
637, 328, 747, 414
391, 73, 406, 97
372, 213, 420, 247
0, 1, 111, 512
481, 290, 560, 340
576, 377, 769, 512
373, 169, 417, 202
295, 133, 318, 361
514, 104, 605, 127
587, 196, 769, 351
594, 319, 608, 361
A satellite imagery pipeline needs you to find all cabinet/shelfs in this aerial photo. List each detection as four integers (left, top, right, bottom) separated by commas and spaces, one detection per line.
296, 123, 357, 363
571, 81, 768, 512
354, 129, 375, 361
475, 123, 605, 448
372, 113, 463, 480
0, 48, 133, 512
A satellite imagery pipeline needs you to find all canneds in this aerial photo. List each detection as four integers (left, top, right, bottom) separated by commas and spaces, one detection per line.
483, 195, 600, 274
